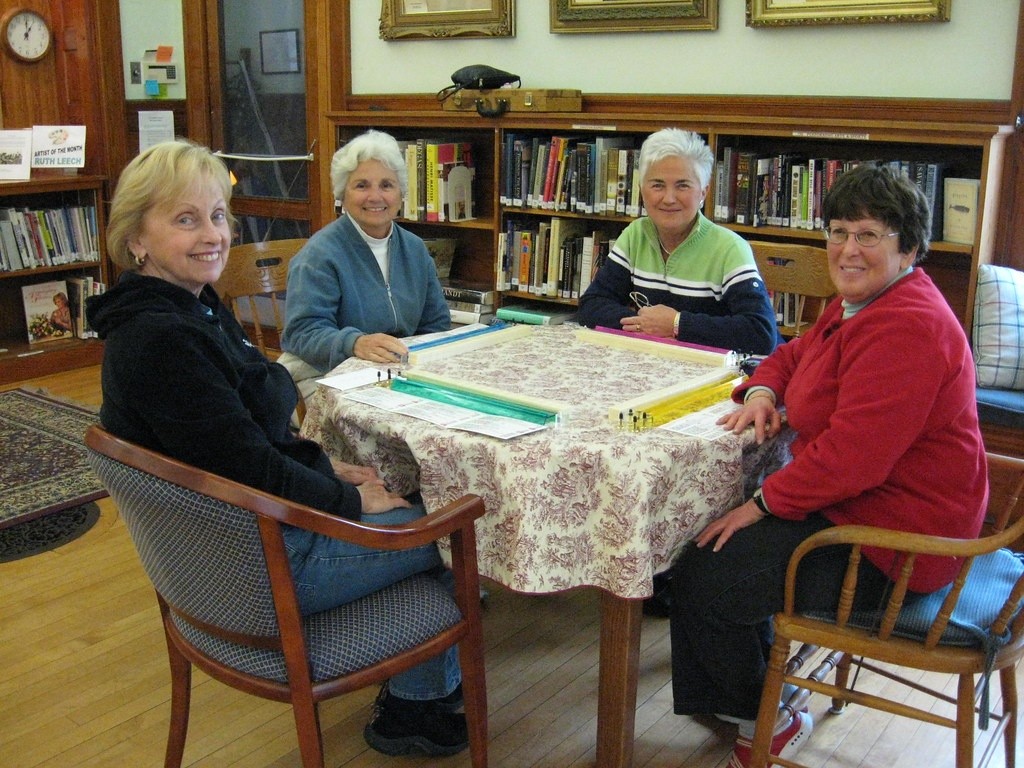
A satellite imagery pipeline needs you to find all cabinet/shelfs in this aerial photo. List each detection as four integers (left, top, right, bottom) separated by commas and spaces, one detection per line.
0, 173, 116, 386
326, 113, 1014, 340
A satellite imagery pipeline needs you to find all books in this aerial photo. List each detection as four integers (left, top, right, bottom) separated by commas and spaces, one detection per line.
0, 188, 100, 272
715, 145, 981, 245
22, 276, 106, 344
396, 140, 478, 222
767, 258, 794, 324
497, 132, 650, 218
497, 306, 579, 325
422, 238, 495, 324
496, 216, 617, 298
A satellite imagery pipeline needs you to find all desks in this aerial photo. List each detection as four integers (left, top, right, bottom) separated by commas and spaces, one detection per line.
297, 323, 797, 768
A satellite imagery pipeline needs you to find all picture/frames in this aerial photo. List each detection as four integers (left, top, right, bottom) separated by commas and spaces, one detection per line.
259, 29, 300, 74
548, 0, 720, 34
746, 0, 953, 30
379, 0, 517, 41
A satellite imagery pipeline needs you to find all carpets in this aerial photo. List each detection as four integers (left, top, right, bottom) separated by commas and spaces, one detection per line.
0, 500, 101, 564
0, 387, 109, 529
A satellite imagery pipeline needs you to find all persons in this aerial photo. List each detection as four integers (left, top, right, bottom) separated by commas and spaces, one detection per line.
93, 141, 468, 757
669, 161, 989, 767
51, 292, 71, 331
579, 127, 786, 354
280, 130, 487, 600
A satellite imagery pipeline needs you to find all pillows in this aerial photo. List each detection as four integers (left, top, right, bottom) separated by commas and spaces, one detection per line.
972, 264, 1023, 391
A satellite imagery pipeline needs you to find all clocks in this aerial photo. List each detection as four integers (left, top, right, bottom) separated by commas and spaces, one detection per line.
0, 7, 53, 63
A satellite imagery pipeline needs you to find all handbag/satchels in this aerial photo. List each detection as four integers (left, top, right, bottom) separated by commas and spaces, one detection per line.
437, 64, 520, 101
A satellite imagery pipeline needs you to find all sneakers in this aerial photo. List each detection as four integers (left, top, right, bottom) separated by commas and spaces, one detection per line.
725, 711, 813, 768
713, 700, 808, 724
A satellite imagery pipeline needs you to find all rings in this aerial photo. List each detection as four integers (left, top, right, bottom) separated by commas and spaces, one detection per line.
385, 486, 391, 492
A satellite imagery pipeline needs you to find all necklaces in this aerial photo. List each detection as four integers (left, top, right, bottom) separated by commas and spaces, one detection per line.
659, 238, 671, 255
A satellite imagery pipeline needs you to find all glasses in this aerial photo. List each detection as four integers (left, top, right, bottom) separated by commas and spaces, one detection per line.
629, 291, 652, 309
823, 226, 902, 247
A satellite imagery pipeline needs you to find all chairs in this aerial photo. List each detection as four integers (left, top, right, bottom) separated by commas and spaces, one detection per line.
974, 387, 1024, 553
750, 452, 1024, 768
750, 240, 835, 341
84, 425, 489, 768
210, 236, 309, 435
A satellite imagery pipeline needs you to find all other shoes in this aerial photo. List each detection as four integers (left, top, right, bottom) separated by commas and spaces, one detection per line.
363, 681, 469, 757
420, 683, 465, 712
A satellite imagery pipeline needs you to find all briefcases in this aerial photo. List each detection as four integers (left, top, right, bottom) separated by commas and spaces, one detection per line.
443, 89, 583, 119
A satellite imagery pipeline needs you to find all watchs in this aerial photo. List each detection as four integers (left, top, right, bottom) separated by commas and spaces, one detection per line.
753, 487, 771, 515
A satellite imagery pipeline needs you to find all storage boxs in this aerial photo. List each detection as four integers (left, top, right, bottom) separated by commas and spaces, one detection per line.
443, 88, 583, 112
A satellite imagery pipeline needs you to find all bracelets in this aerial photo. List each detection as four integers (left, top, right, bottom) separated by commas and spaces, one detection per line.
746, 394, 774, 403
